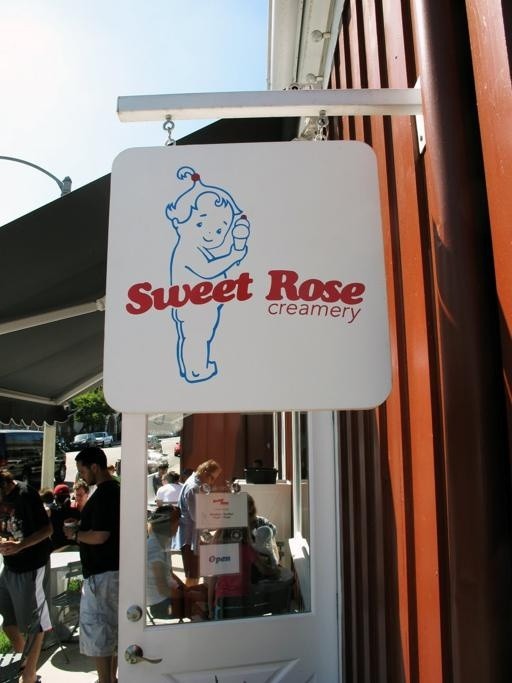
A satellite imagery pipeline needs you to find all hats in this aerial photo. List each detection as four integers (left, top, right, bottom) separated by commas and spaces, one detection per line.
53, 484, 70, 497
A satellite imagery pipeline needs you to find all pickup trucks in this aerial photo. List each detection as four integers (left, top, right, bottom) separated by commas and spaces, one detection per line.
96, 432, 113, 447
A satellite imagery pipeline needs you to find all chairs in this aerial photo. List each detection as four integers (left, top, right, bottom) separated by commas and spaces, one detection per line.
1, 558, 84, 683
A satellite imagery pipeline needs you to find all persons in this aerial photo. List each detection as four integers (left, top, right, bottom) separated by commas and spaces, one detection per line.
2, 470, 53, 681
42, 472, 89, 552
63, 450, 119, 681
146, 447, 292, 619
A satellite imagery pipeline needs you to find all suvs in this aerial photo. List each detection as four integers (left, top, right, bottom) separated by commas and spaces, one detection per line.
69, 433, 97, 450
1, 429, 66, 485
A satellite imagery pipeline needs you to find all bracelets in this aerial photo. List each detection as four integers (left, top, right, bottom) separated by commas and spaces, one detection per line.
72, 530, 79, 542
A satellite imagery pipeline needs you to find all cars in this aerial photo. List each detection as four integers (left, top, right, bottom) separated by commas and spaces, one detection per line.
174, 442, 181, 455
148, 435, 161, 453
